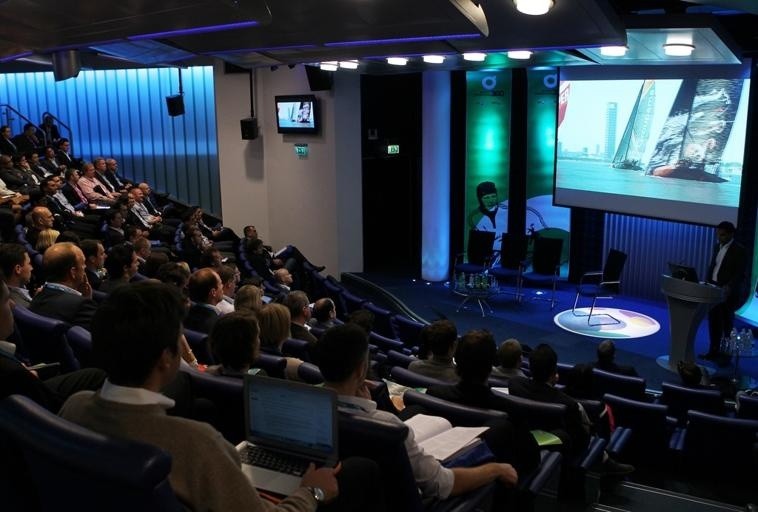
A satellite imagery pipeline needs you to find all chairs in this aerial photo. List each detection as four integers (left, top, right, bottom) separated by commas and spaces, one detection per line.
589, 367, 646, 402
322, 280, 342, 312
312, 270, 324, 291
297, 363, 391, 417
91, 288, 109, 302
282, 338, 309, 357
401, 388, 505, 448
604, 394, 668, 471
180, 368, 245, 436
661, 382, 726, 421
175, 221, 185, 254
303, 262, 313, 279
454, 228, 496, 289
338, 410, 501, 512
368, 331, 403, 353
519, 237, 564, 311
256, 354, 288, 380
491, 387, 604, 475
385, 350, 417, 372
10, 301, 78, 374
67, 325, 93, 370
391, 366, 458, 394
235, 241, 263, 282
685, 409, 758, 472
491, 232, 522, 305
737, 395, 758, 416
571, 249, 628, 326
183, 328, 209, 364
487, 376, 510, 387
364, 302, 390, 333
263, 281, 280, 297
15, 224, 45, 266
394, 316, 424, 342
402, 390, 561, 500
2, 393, 172, 502
340, 291, 364, 318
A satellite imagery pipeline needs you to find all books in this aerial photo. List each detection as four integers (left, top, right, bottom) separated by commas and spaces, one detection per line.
405, 413, 490, 463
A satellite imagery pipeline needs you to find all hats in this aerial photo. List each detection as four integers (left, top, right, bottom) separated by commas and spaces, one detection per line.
476, 182, 498, 200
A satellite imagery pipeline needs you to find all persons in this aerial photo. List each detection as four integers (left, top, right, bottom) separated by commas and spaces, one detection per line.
236, 277, 273, 305
159, 263, 190, 291
468, 181, 507, 268
11, 151, 45, 190
201, 248, 222, 267
201, 313, 266, 381
49, 175, 100, 226
180, 227, 235, 268
183, 267, 223, 334
316, 324, 516, 502
26, 151, 54, 179
79, 162, 118, 205
55, 283, 342, 512
247, 237, 302, 289
143, 253, 169, 279
191, 205, 240, 244
0, 271, 107, 414
63, 169, 111, 215
243, 226, 326, 272
233, 285, 263, 319
40, 179, 98, 237
80, 239, 107, 290
509, 344, 633, 475
273, 268, 294, 294
56, 229, 80, 246
129, 187, 181, 244
0, 178, 30, 214
597, 339, 637, 377
124, 224, 142, 245
282, 289, 318, 349
347, 309, 385, 355
667, 360, 714, 391
698, 221, 751, 368
409, 320, 460, 386
0, 156, 35, 195
100, 244, 141, 298
0, 243, 36, 309
181, 211, 237, 252
34, 229, 60, 255
45, 147, 70, 180
111, 202, 177, 261
308, 299, 339, 329
427, 327, 540, 471
104, 210, 125, 247
135, 237, 190, 280
0, 208, 18, 242
254, 303, 293, 355
138, 182, 186, 220
225, 261, 242, 298
24, 205, 56, 249
29, 243, 102, 330
735, 387, 758, 412
56, 138, 83, 174
213, 265, 238, 314
35, 116, 62, 146
407, 325, 432, 362
29, 190, 94, 241
94, 158, 122, 200
120, 192, 175, 244
16, 125, 38, 159
106, 159, 136, 190
0, 126, 19, 164
490, 338, 529, 384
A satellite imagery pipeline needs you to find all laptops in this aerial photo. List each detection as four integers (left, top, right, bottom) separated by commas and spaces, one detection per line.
235, 372, 340, 497
668, 263, 698, 285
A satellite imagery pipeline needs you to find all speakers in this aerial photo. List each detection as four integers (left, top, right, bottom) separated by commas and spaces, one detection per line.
304, 64, 332, 91
166, 95, 185, 116
241, 118, 258, 140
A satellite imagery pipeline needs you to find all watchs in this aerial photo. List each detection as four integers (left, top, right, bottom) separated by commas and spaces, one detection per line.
306, 486, 326, 506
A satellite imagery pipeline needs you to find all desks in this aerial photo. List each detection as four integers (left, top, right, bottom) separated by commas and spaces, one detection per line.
454, 285, 497, 318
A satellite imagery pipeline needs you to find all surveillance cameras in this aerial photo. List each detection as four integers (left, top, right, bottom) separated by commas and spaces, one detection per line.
270, 66, 278, 71
288, 64, 294, 68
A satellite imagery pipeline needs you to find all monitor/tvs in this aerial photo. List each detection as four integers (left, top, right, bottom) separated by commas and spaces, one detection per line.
274, 94, 320, 135
